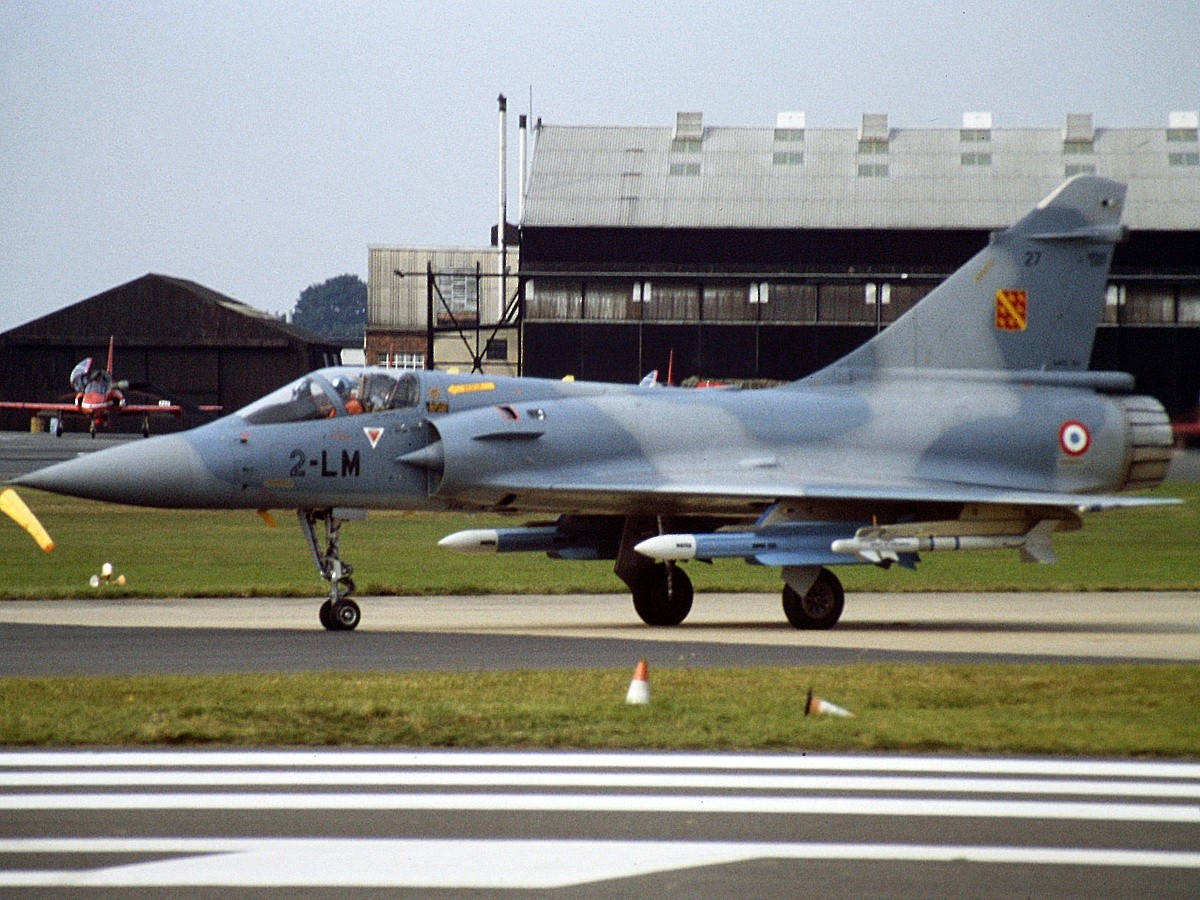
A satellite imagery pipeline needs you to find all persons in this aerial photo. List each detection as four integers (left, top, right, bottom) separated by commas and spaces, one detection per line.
320, 377, 365, 419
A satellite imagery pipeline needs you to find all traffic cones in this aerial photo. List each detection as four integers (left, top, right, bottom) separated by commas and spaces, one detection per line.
625, 661, 651, 705
803, 686, 858, 719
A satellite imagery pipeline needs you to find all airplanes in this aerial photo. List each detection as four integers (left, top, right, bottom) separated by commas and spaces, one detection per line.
1, 333, 225, 440
0, 171, 1174, 633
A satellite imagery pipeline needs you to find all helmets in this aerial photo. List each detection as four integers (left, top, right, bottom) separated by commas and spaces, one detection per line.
332, 376, 351, 397
